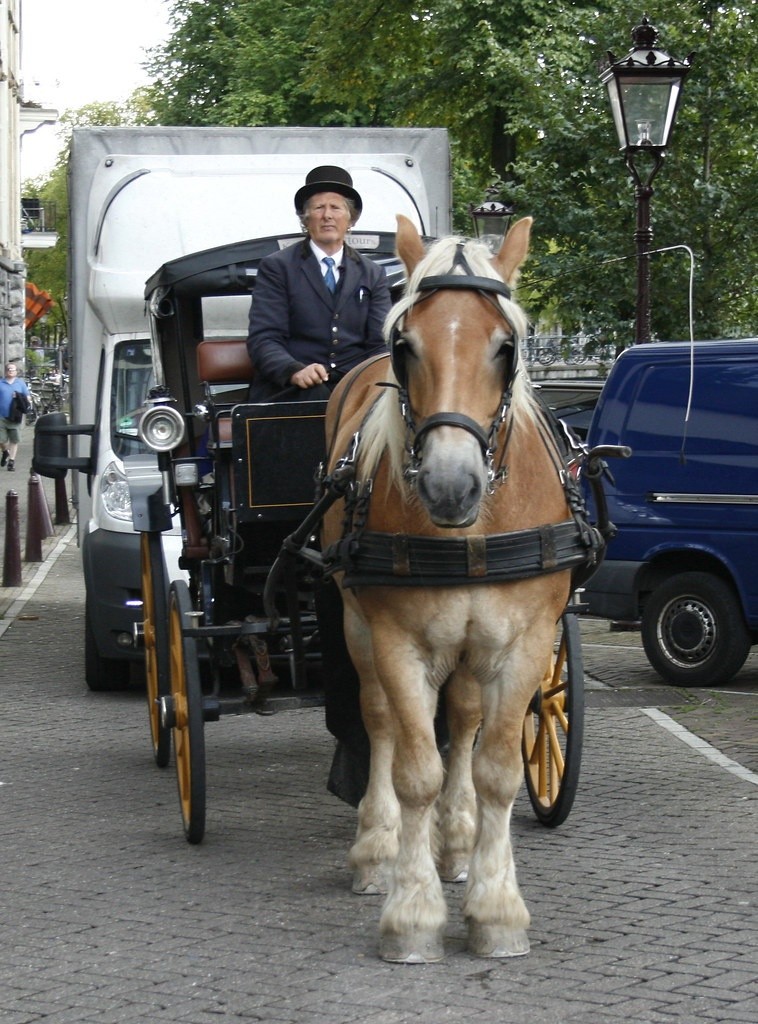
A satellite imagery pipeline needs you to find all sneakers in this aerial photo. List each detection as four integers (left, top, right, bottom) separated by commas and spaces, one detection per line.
8, 459, 15, 471
1, 451, 9, 467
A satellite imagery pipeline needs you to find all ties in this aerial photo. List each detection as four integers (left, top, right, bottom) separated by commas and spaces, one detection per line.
322, 257, 337, 296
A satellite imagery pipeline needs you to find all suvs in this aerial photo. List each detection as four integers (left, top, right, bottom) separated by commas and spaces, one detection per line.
572, 339, 757, 688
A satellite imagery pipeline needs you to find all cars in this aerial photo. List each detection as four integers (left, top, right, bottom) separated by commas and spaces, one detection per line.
529, 379, 606, 442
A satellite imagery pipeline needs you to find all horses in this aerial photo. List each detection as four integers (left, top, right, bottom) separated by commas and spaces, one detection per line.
319, 213, 577, 965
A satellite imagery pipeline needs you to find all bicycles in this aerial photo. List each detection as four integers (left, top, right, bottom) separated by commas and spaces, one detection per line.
24, 369, 69, 426
521, 332, 617, 365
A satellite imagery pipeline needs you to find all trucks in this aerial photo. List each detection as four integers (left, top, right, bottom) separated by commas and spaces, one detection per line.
30, 126, 451, 691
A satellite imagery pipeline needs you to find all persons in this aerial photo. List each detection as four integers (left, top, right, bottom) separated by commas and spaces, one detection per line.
0, 362, 31, 472
247, 165, 394, 405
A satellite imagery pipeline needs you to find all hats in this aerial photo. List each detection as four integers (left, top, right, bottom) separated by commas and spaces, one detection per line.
31, 336, 40, 342
294, 165, 363, 226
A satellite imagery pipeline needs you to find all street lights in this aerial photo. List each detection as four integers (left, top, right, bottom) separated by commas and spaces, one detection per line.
596, 15, 691, 347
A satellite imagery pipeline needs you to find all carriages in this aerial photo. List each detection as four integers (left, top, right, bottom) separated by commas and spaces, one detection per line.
124, 215, 634, 965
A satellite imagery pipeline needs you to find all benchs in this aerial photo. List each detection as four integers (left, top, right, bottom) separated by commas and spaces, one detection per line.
196, 338, 256, 457
